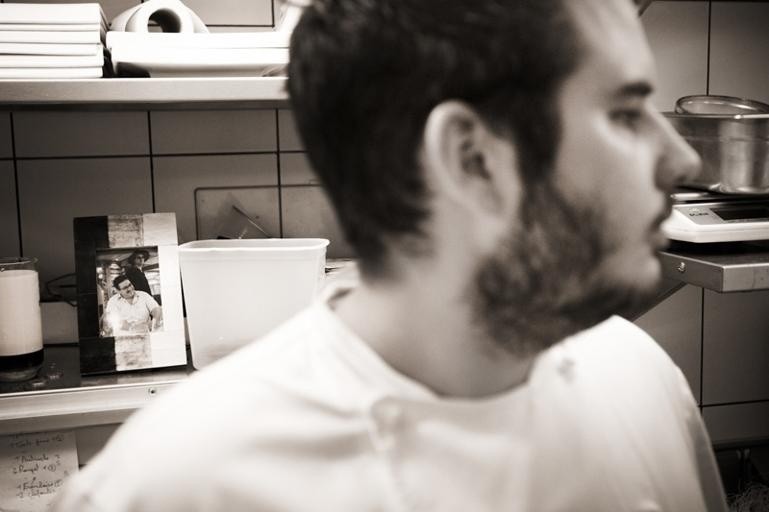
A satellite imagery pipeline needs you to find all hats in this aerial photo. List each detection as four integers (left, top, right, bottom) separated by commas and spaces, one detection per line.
128, 249, 148, 264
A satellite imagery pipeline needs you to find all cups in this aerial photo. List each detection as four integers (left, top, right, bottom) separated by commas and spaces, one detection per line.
0, 253, 47, 382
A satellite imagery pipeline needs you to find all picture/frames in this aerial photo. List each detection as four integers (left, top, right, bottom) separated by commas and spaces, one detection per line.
69, 210, 190, 377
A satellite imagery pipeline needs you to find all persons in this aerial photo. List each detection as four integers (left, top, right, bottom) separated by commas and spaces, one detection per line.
45, 0, 731, 512
125, 248, 153, 297
99, 274, 162, 338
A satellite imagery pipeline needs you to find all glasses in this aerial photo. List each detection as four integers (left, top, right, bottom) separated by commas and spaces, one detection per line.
137, 256, 145, 259
